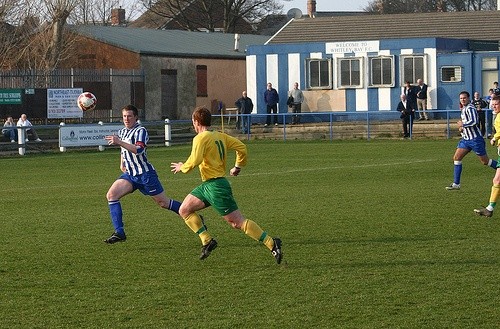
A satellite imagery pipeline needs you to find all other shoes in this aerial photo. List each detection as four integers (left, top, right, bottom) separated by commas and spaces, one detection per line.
426, 117, 430, 120
482, 131, 485, 136
488, 134, 492, 138
26, 139, 29, 142
403, 134, 408, 138
292, 122, 295, 124
35, 138, 42, 142
275, 123, 278, 125
419, 118, 424, 120
11, 140, 15, 143
264, 124, 267, 127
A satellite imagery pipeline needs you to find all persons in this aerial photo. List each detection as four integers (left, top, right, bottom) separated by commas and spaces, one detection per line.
444, 91, 499, 190
264, 83, 280, 126
234, 91, 254, 134
415, 79, 430, 121
288, 82, 305, 125
1, 117, 20, 142
402, 80, 417, 120
169, 105, 285, 266
472, 80, 500, 140
396, 93, 415, 138
102, 103, 205, 242
472, 94, 500, 216
17, 114, 43, 143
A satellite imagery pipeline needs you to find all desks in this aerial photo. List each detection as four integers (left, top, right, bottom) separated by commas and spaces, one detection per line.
226, 108, 239, 124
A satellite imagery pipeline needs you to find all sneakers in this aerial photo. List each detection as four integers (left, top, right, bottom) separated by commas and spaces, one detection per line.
199, 215, 208, 230
474, 206, 493, 217
445, 183, 460, 190
271, 238, 283, 264
104, 233, 126, 244
199, 239, 217, 260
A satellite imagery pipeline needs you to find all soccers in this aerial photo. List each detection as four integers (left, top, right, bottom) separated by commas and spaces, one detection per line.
77, 91, 97, 111
